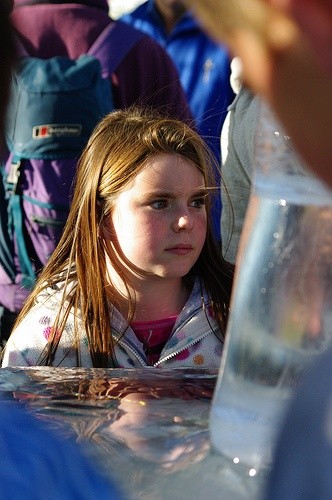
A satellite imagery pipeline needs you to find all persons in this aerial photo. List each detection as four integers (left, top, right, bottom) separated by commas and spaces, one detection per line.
0, 0, 199, 352
0, 110, 237, 371
113, 0, 238, 301
30, 368, 215, 500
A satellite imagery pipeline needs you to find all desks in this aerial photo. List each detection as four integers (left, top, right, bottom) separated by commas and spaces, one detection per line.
0, 365, 269, 500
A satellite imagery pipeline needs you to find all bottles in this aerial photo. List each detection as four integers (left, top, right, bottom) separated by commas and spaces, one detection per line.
208, 99, 332, 472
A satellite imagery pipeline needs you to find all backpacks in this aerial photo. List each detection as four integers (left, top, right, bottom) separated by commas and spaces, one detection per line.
0, 20, 145, 312
